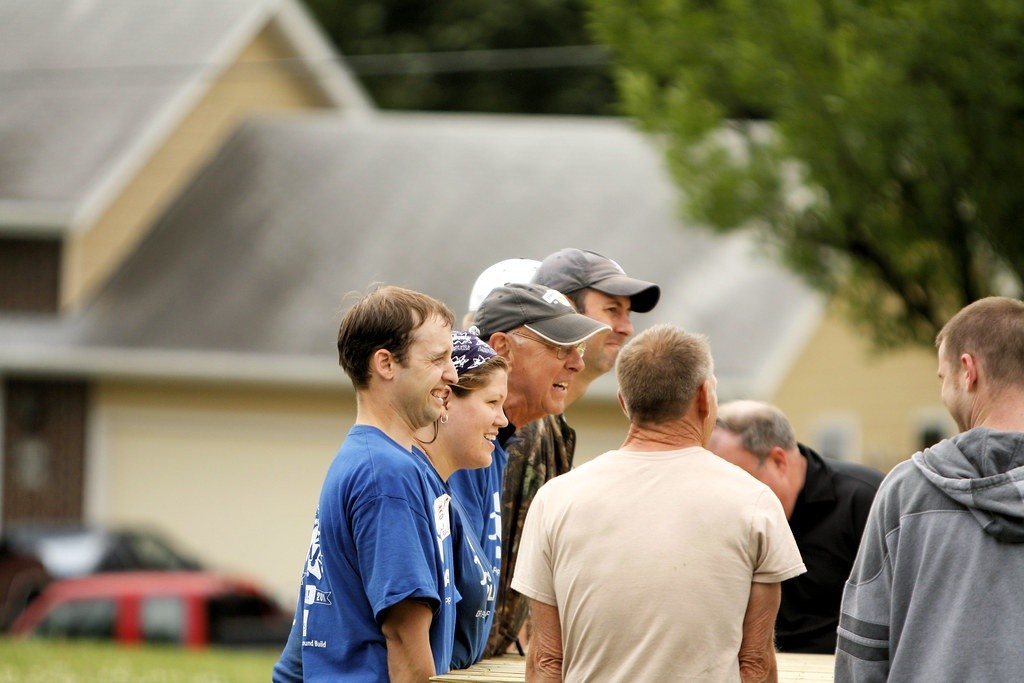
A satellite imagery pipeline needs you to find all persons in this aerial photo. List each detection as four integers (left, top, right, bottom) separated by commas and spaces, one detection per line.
709, 401, 886, 653
834, 294, 1024, 682
510, 323, 809, 683
447, 247, 661, 670
268, 331, 509, 683
297, 286, 460, 683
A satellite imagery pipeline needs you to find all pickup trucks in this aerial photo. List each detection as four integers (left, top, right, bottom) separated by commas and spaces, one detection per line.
4, 571, 294, 649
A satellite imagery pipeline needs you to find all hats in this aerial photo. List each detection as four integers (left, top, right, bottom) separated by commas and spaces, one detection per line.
472, 283, 614, 345
536, 248, 661, 314
469, 258, 542, 312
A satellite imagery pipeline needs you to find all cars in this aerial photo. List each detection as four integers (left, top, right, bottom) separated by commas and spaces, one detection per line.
0, 529, 203, 635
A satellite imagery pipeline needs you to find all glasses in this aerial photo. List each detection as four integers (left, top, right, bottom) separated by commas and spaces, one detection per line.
512, 330, 588, 361
